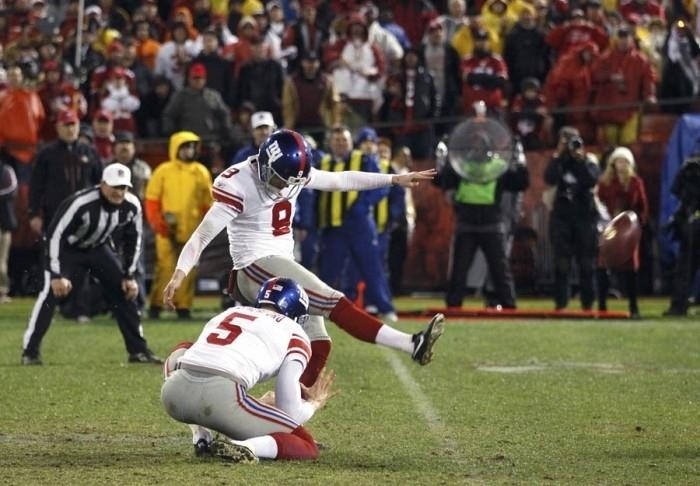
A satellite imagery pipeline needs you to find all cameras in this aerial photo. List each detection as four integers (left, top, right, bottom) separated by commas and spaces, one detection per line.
568, 136, 583, 150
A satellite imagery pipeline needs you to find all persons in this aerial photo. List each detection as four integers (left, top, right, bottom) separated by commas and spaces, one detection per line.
1, 0, 700, 322
163, 129, 448, 400
159, 276, 341, 463
22, 162, 163, 366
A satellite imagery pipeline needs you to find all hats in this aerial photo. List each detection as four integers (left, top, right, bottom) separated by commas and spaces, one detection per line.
354, 127, 381, 145
102, 162, 136, 188
250, 112, 277, 128
94, 111, 112, 121
2, 5, 698, 110
57, 110, 79, 126
607, 144, 636, 165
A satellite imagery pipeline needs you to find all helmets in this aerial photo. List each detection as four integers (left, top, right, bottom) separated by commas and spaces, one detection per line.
255, 276, 310, 325
257, 127, 313, 203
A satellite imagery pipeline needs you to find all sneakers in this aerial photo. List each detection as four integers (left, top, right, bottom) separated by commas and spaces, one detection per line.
408, 312, 445, 366
193, 437, 212, 458
127, 349, 166, 365
21, 351, 44, 366
209, 440, 260, 465
